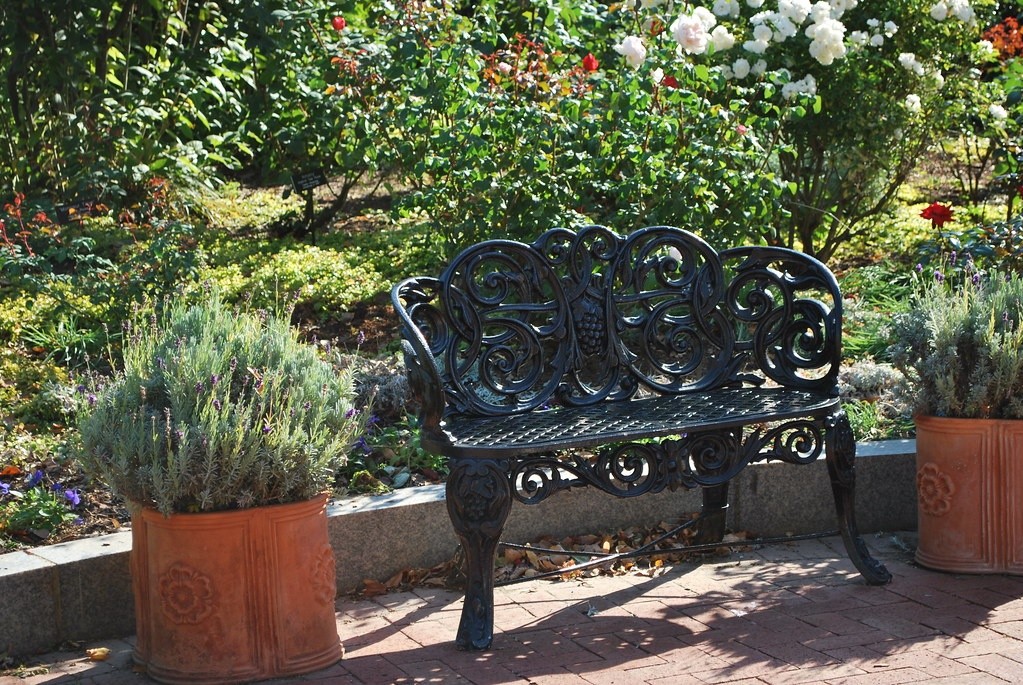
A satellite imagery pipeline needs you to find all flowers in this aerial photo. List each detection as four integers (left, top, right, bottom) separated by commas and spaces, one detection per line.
874, 251, 1023, 418
49, 273, 377, 524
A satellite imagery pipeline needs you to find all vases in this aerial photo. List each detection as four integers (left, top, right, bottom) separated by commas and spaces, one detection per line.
912, 411, 1023, 576
125, 496, 346, 685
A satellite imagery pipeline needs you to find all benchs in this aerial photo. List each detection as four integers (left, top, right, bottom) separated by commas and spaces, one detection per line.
391, 224, 892, 653
